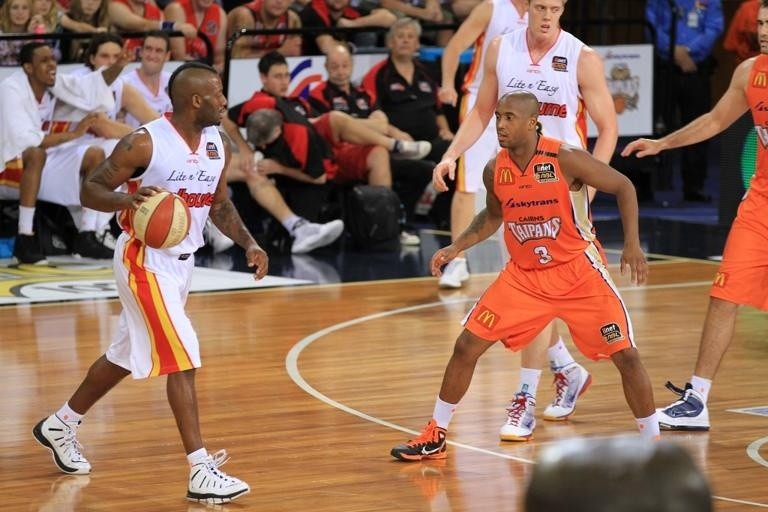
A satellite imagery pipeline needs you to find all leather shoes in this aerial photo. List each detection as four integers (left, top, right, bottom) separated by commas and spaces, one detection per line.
654, 181, 674, 191
683, 190, 711, 201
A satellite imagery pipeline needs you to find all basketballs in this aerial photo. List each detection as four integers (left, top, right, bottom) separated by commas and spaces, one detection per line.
131, 191, 191, 248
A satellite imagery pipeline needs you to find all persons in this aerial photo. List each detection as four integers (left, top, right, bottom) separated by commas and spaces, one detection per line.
32, 61, 271, 504
523, 434, 713, 511
437, 1, 530, 288
620, 0, 768, 432
718, 0, 764, 224
390, 90, 660, 461
0, 0, 484, 254
644, 0, 724, 203
430, 1, 620, 444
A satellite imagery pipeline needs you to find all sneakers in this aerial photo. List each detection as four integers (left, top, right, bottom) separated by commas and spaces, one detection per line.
389, 138, 433, 162
499, 383, 536, 442
288, 217, 344, 255
186, 450, 252, 506
438, 255, 472, 289
13, 232, 46, 264
541, 359, 592, 422
655, 381, 711, 431
398, 229, 421, 247
203, 215, 236, 256
96, 224, 118, 249
70, 230, 114, 260
31, 412, 93, 477
389, 418, 448, 462
